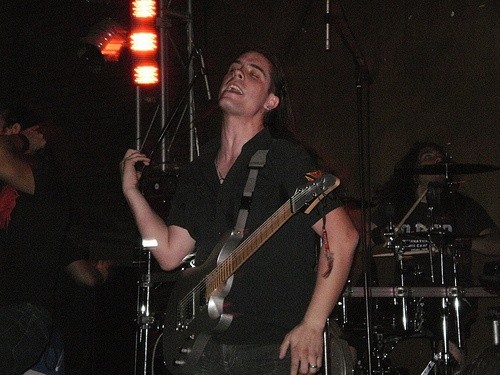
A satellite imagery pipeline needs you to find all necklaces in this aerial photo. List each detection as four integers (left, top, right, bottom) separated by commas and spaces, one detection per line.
216, 155, 225, 184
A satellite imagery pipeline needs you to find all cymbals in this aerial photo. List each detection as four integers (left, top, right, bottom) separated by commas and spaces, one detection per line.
398, 228, 488, 243
404, 164, 500, 175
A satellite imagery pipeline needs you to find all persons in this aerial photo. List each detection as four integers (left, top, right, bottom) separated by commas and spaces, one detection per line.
118, 43, 360, 375
330, 138, 496, 375
1, 96, 114, 375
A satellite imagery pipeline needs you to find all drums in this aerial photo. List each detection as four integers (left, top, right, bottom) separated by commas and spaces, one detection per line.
436, 306, 469, 375
325, 308, 371, 375
372, 304, 436, 375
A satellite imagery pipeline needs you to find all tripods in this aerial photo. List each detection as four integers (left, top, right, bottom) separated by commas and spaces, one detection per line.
419, 241, 459, 375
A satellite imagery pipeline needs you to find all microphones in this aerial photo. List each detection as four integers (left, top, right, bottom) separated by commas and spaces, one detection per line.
325, 0, 330, 51
199, 48, 212, 100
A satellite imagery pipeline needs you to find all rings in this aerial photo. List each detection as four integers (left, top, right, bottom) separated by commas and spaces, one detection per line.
309, 365, 318, 368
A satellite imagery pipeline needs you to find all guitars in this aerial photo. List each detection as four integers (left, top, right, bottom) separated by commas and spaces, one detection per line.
162, 174, 339, 375
